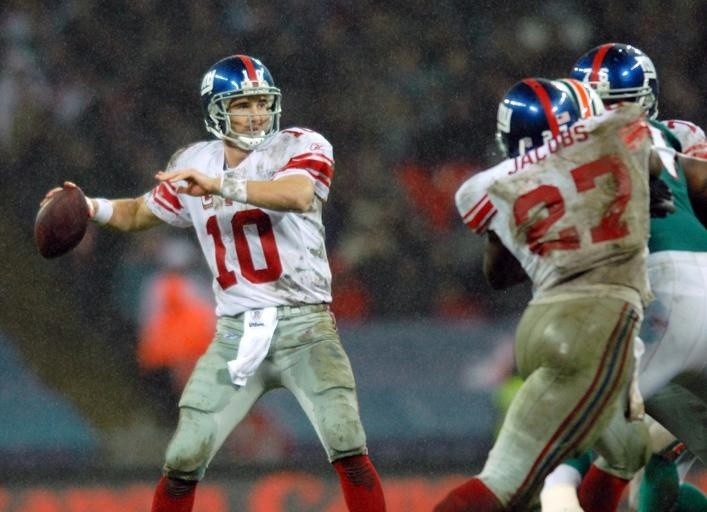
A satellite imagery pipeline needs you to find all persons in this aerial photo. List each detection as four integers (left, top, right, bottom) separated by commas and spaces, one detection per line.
39, 55, 386, 512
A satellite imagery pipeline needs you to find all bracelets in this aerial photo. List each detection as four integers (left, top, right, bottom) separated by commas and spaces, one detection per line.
88, 197, 113, 224
219, 177, 247, 203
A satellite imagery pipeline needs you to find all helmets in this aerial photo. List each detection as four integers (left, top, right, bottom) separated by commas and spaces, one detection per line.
493, 43, 661, 161
198, 55, 283, 152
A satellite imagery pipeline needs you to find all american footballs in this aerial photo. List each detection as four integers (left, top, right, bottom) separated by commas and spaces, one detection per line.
36, 185, 90, 259
